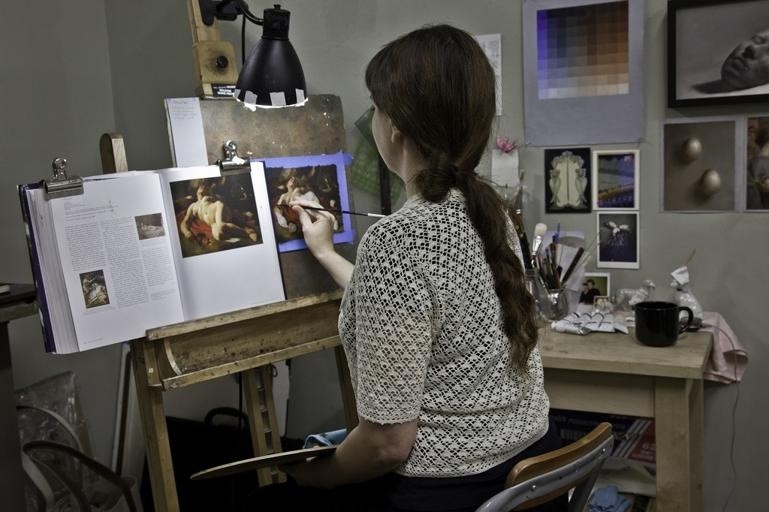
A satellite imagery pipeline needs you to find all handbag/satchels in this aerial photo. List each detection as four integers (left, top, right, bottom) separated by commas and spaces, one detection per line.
140, 406, 305, 511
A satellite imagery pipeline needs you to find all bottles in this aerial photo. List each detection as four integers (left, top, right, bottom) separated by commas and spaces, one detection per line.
524, 268, 553, 331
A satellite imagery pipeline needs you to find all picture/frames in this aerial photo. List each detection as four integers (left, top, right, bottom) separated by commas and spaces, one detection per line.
580, 272, 610, 305
666, 1, 767, 110
594, 149, 642, 270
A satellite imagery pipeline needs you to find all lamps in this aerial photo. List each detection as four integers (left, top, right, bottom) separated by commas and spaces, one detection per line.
199, 0, 309, 110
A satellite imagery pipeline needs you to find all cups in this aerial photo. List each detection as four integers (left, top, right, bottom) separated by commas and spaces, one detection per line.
543, 289, 569, 321
634, 300, 695, 348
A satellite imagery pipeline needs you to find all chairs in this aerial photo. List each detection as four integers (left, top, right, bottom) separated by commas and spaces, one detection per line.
475, 422, 618, 512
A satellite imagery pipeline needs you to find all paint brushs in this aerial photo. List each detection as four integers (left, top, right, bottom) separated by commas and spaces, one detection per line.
284, 204, 386, 218
531, 220, 586, 318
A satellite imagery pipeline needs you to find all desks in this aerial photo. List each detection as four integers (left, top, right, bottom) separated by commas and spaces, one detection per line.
526, 321, 714, 510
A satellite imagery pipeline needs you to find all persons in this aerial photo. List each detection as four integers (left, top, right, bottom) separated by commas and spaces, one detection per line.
180, 182, 256, 248
584, 280, 601, 304
139, 221, 162, 232
274, 175, 340, 233
82, 274, 109, 307
220, 22, 568, 510
719, 27, 769, 92
578, 283, 589, 303
